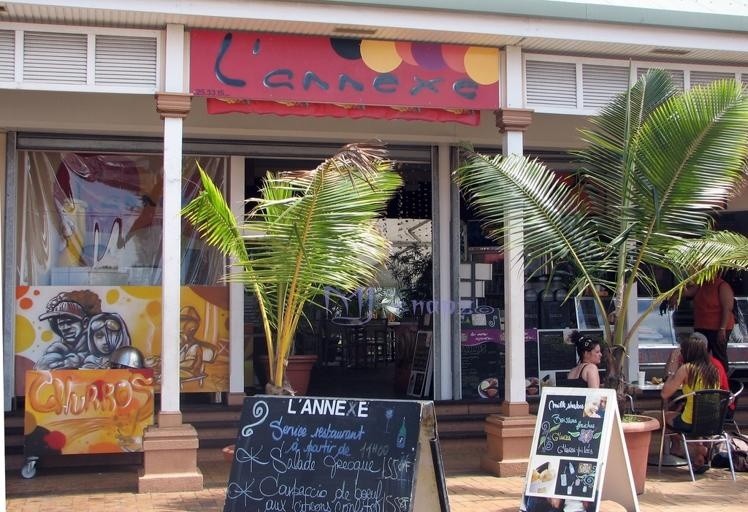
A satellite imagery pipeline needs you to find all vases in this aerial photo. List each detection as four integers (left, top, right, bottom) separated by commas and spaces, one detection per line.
256, 351, 317, 396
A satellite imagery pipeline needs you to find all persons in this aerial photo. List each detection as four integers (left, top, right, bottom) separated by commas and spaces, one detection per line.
30, 300, 90, 370
565, 330, 603, 387
79, 312, 133, 370
668, 268, 735, 373
664, 332, 736, 458
660, 333, 721, 474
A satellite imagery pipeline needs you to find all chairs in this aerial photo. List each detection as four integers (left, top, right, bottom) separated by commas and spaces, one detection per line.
659, 389, 735, 482
722, 377, 744, 434
356, 318, 388, 367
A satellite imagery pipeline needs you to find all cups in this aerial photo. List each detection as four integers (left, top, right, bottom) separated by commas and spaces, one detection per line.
637, 371, 646, 385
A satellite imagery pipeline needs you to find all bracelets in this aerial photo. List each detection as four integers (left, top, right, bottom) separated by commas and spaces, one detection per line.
720, 327, 726, 330
669, 358, 680, 364
667, 372, 675, 375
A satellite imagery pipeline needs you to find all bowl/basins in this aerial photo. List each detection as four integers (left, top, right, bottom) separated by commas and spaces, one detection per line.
106, 345, 143, 369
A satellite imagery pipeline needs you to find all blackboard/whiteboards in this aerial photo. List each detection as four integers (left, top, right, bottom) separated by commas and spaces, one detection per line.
223, 396, 421, 512
535, 386, 615, 503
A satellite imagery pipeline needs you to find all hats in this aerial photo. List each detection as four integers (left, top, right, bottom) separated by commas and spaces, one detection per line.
39, 301, 86, 322
180, 306, 200, 321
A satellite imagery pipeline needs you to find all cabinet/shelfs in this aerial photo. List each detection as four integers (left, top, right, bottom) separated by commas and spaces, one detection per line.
574, 295, 748, 382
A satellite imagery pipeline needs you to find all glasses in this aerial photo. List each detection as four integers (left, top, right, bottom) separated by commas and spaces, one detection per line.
90, 319, 123, 332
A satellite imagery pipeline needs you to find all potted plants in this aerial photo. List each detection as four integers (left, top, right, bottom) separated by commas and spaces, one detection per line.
453, 68, 748, 494
182, 136, 406, 469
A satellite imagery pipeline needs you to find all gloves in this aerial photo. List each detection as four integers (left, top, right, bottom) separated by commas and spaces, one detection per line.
660, 296, 668, 316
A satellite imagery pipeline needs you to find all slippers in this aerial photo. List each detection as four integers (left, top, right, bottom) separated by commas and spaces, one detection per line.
692, 462, 710, 474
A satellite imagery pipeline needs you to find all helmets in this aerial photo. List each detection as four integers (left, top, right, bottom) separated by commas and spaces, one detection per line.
111, 347, 145, 370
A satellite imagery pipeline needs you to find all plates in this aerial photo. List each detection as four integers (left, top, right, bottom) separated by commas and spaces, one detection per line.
478, 378, 499, 398
